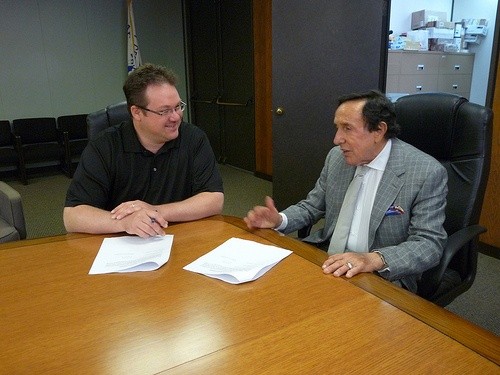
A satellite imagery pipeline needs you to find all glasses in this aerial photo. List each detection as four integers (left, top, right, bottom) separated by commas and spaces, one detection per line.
133, 100, 186, 116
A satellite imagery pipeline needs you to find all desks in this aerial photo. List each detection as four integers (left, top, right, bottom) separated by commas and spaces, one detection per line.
0, 214, 500, 375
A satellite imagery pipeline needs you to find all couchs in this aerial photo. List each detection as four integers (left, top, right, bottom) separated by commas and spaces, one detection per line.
0, 180, 27, 242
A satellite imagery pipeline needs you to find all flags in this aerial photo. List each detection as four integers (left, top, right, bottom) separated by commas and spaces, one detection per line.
127, 0, 143, 76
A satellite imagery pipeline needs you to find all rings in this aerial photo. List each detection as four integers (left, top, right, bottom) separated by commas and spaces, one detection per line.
131, 204, 135, 207
346, 262, 353, 269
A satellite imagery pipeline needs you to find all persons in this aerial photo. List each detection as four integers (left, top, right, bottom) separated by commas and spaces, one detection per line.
63, 63, 225, 239
243, 89, 449, 293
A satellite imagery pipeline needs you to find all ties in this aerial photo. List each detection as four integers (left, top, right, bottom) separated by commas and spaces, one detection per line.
327, 167, 366, 257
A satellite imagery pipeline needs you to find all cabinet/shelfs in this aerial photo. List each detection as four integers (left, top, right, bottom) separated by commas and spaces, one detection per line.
386, 48, 475, 99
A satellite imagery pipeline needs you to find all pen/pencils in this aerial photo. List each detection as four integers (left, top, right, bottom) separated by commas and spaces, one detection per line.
151, 209, 158, 223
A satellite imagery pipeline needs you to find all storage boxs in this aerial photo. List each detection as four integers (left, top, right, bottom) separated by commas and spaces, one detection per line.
388, 10, 489, 53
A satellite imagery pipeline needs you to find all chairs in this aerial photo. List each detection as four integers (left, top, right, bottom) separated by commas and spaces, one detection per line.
0, 101, 131, 184
297, 92, 495, 308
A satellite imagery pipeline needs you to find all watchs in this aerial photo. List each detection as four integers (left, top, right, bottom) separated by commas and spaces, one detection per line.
378, 252, 385, 265
